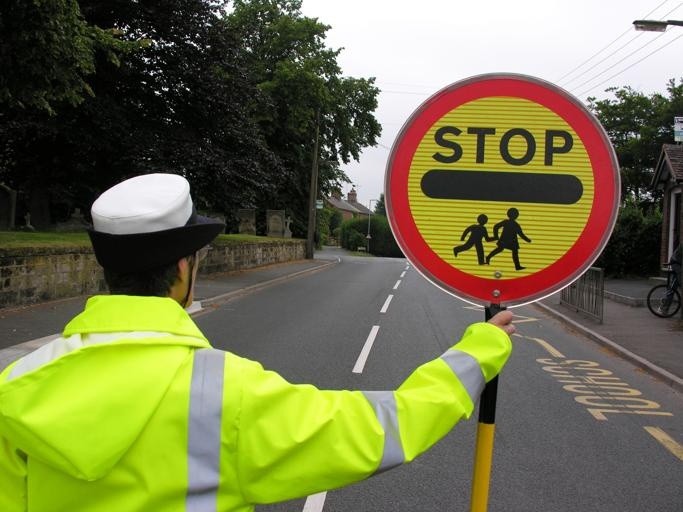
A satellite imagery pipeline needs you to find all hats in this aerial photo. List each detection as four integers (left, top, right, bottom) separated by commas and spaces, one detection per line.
85, 173, 225, 265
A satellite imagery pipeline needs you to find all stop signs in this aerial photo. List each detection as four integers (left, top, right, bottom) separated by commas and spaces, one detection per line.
383, 72, 622, 310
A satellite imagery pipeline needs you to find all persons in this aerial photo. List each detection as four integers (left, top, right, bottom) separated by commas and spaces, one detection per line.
0, 172, 515, 512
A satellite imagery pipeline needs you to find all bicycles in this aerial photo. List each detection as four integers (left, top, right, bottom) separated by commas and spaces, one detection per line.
647, 262, 683, 318
